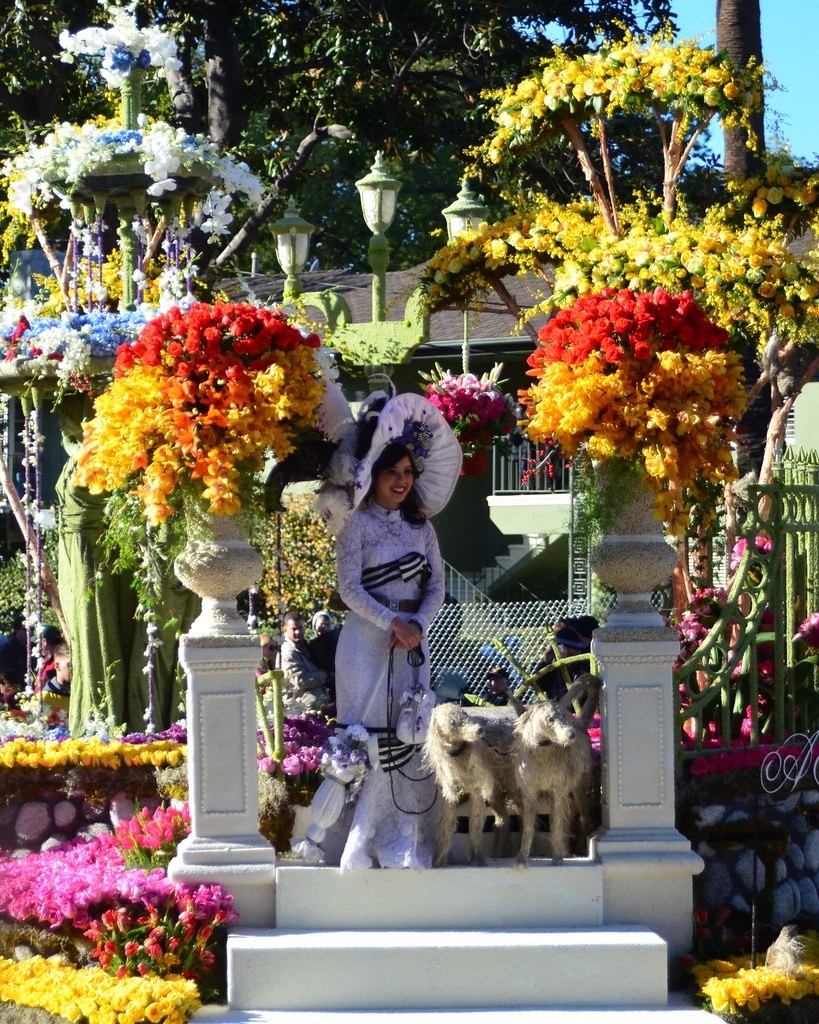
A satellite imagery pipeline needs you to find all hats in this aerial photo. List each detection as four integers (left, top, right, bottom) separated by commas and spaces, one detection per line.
312, 611, 332, 632
487, 667, 509, 680
349, 392, 462, 518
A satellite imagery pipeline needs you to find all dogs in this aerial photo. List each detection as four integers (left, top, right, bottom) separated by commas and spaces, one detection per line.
416, 697, 598, 870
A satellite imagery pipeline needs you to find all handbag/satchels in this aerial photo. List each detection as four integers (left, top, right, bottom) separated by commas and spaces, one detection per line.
396, 648, 436, 744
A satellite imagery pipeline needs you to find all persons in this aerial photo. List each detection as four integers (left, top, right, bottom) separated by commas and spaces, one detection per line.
481, 668, 515, 705
277, 613, 328, 707
0, 617, 36, 685
308, 602, 339, 673
533, 615, 597, 698
29, 625, 66, 691
42, 643, 73, 695
328, 440, 446, 871
